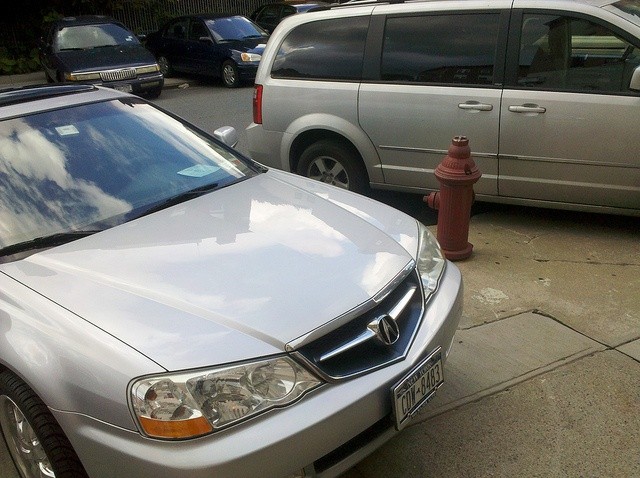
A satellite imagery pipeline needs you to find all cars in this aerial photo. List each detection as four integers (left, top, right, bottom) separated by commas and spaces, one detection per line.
144, 13, 270, 88
38, 16, 165, 99
0, 83, 464, 478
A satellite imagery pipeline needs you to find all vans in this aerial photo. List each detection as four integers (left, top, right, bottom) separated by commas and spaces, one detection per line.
245, 0, 640, 217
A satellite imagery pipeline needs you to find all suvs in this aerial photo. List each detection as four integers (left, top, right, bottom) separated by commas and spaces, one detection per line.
250, 3, 332, 34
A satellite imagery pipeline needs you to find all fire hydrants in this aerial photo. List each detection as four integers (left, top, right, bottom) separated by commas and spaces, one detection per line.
422, 135, 483, 263
